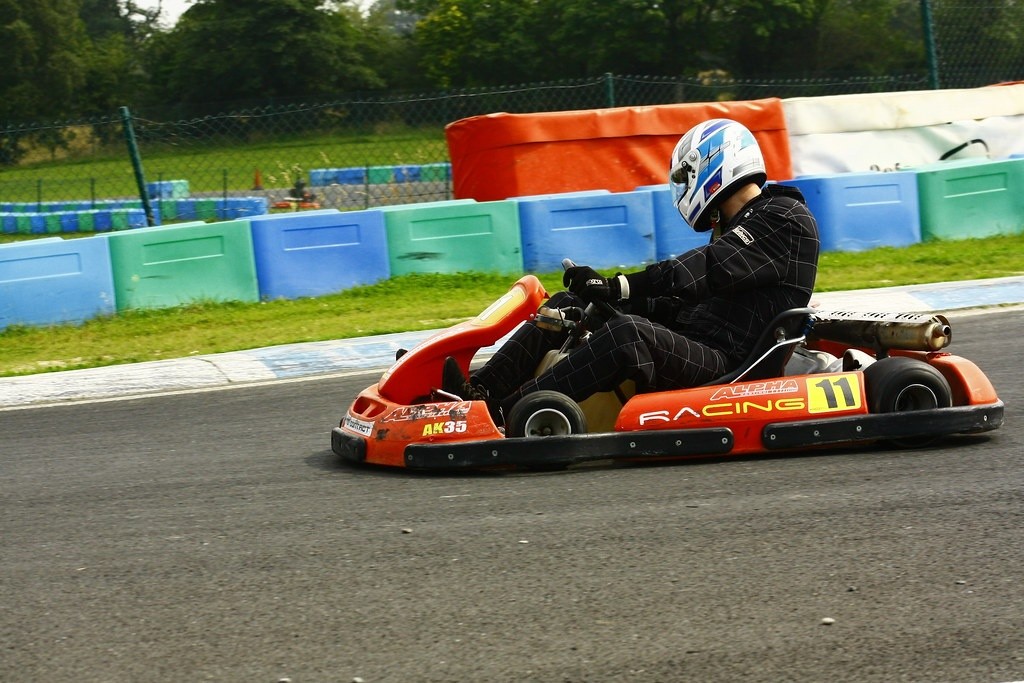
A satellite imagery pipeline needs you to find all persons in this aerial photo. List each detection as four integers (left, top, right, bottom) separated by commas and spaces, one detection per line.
398, 117, 820, 428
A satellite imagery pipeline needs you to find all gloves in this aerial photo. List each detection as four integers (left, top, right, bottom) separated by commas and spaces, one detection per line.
562, 265, 622, 305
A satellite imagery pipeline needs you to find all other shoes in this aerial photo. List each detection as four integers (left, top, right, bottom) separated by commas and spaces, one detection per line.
396, 350, 409, 360
442, 356, 487, 403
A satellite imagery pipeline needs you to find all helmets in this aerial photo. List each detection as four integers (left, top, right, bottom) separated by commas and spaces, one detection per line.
669, 118, 769, 232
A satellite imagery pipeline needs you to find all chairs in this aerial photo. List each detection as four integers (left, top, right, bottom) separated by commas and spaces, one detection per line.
697, 307, 817, 388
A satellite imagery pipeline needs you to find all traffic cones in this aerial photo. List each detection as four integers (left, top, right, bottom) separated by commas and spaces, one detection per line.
251, 171, 264, 190
